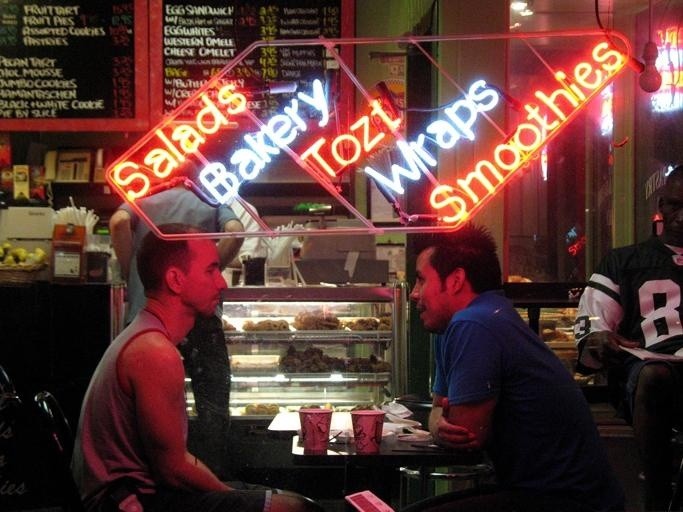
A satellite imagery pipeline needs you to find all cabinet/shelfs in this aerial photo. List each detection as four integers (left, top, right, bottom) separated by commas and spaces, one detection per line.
505, 282, 610, 401
117, 284, 406, 426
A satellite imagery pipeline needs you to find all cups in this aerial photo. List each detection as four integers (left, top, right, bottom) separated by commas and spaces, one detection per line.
245, 261, 264, 285
86, 255, 107, 281
300, 408, 386, 454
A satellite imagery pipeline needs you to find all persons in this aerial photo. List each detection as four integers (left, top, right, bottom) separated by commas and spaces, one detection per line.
400, 225, 620, 512
228, 198, 259, 269
70, 223, 303, 512
575, 164, 683, 512
109, 160, 244, 473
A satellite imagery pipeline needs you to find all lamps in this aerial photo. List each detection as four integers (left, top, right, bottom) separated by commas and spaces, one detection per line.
640, 1, 661, 91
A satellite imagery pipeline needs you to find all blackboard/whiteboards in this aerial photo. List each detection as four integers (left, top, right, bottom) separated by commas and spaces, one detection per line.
1, 2, 148, 131
150, 4, 355, 130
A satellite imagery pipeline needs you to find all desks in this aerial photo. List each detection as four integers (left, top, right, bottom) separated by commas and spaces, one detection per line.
268, 431, 484, 512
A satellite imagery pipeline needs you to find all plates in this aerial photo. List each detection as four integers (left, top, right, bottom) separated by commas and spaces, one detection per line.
333, 431, 434, 446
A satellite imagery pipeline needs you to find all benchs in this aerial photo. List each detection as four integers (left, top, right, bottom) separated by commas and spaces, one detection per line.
589, 404, 683, 511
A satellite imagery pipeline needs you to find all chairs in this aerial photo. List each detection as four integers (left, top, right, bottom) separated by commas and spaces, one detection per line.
33, 392, 80, 510
399, 486, 596, 509
